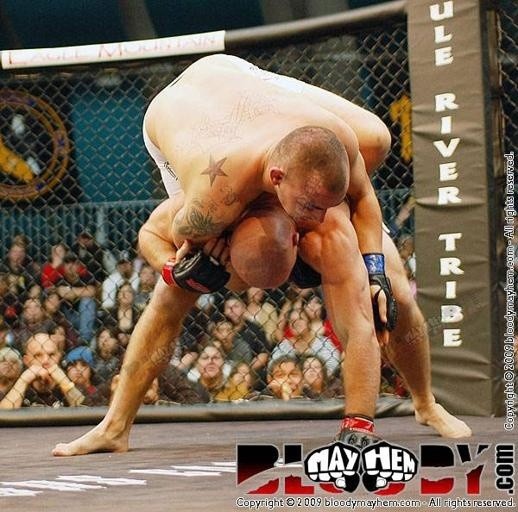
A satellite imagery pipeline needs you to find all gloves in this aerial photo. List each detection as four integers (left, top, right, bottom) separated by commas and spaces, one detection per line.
336, 413, 383, 449
162, 251, 230, 294
362, 253, 398, 331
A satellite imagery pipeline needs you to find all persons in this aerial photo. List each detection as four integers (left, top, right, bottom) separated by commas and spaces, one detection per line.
139, 54, 397, 348
380, 353, 411, 398
51, 190, 471, 458
1, 229, 155, 409
142, 287, 346, 404
386, 201, 416, 300
